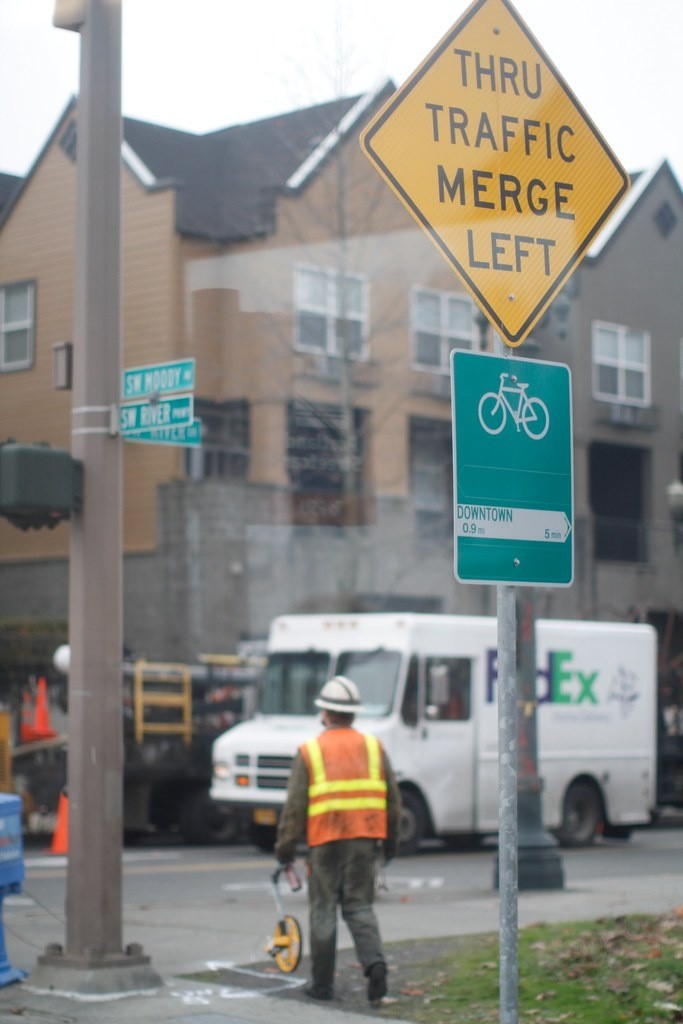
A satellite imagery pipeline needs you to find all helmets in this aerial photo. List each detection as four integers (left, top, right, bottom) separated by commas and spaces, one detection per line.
313, 675, 364, 713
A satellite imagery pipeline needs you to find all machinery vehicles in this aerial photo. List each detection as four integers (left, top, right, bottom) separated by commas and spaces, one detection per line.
1, 601, 264, 837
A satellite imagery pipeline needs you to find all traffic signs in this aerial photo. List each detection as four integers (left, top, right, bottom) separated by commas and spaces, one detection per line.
118, 359, 203, 450
361, 0, 636, 353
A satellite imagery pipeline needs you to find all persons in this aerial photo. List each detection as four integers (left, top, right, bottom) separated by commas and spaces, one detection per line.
274, 674, 402, 1004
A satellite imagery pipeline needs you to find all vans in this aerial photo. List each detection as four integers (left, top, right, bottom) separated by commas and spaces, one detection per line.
199, 591, 662, 855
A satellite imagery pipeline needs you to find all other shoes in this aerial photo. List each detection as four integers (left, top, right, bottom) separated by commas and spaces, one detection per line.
368, 963, 387, 1000
307, 984, 335, 1001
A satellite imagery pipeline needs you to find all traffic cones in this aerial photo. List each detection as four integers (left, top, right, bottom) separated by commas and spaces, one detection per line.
46, 784, 70, 857
